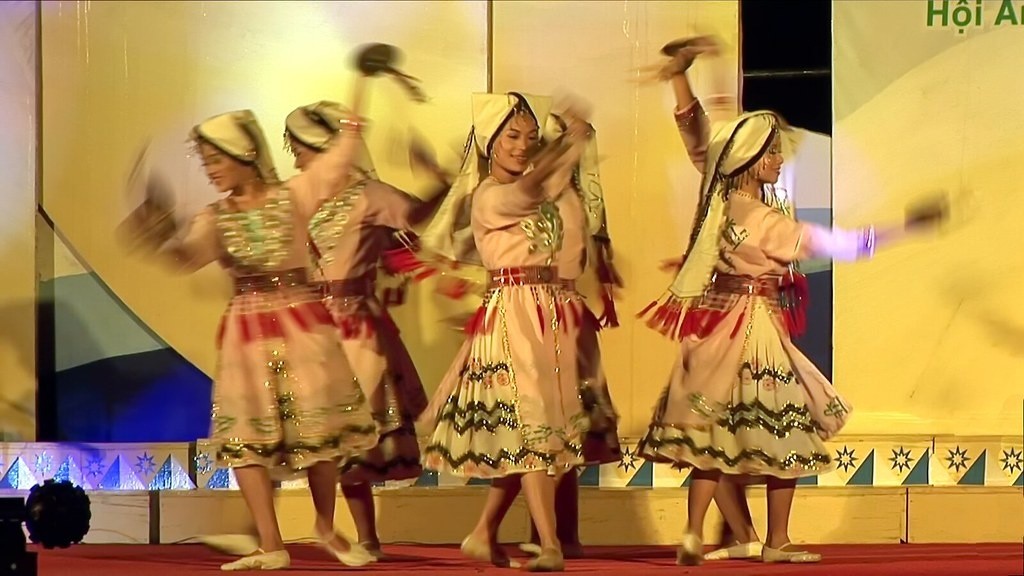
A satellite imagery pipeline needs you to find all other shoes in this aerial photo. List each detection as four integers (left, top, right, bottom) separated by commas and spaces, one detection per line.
462, 535, 521, 568
221, 548, 291, 570
522, 546, 563, 572
704, 540, 762, 559
316, 522, 370, 566
677, 533, 703, 565
762, 542, 822, 563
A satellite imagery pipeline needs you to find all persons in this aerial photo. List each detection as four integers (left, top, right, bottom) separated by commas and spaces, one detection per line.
634, 34, 950, 567
281, 99, 445, 562
409, 91, 622, 572
120, 64, 378, 572
